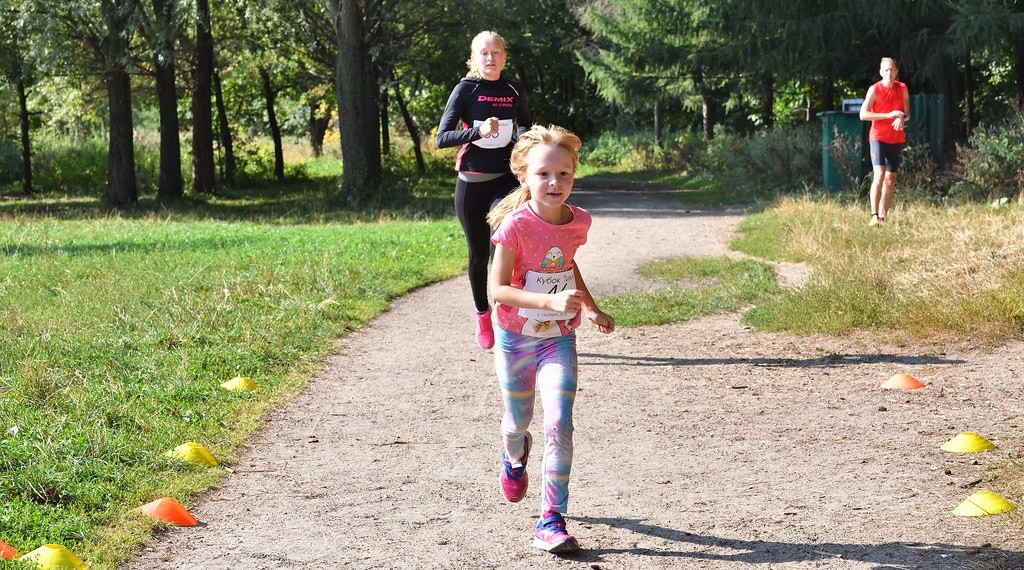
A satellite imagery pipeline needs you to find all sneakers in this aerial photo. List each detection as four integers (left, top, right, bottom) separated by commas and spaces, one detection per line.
500, 431, 532, 502
475, 303, 495, 350
534, 511, 580, 553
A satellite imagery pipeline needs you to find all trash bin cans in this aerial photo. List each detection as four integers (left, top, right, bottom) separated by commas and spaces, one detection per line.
815, 110, 873, 193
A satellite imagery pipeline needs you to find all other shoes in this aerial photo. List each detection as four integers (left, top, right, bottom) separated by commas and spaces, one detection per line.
869, 213, 878, 227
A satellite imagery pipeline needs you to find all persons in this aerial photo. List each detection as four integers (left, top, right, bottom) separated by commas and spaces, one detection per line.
486, 123, 615, 553
437, 31, 525, 350
858, 57, 910, 228
